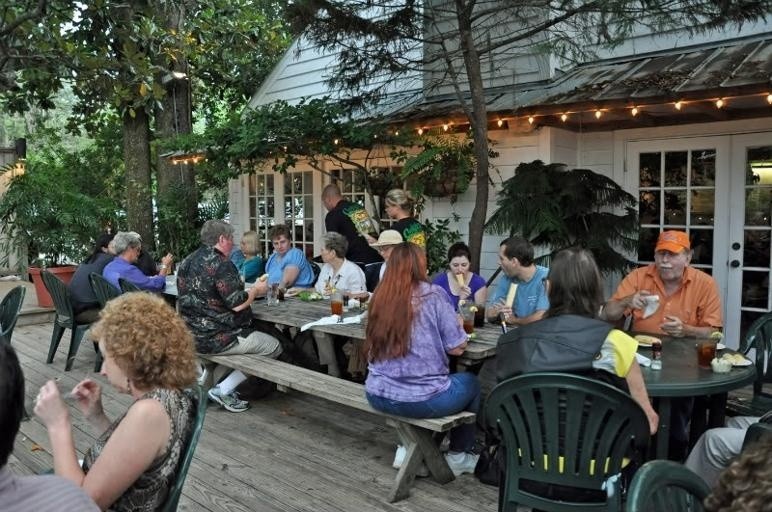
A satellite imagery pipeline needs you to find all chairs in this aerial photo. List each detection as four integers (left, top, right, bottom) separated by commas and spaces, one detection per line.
40, 269, 99, 371
627, 459, 710, 512
483, 374, 650, 512
118, 278, 142, 294
725, 313, 772, 417
88, 272, 122, 372
163, 384, 208, 512
0, 285, 27, 342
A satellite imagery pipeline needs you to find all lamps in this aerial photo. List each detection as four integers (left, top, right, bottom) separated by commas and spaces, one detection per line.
14, 138, 26, 160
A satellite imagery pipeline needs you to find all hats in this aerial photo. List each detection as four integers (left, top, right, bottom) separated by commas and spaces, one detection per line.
370, 229, 403, 246
654, 230, 690, 255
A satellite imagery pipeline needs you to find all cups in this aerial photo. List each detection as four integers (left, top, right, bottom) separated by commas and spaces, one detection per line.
330, 294, 343, 315
697, 336, 716, 369
460, 300, 475, 334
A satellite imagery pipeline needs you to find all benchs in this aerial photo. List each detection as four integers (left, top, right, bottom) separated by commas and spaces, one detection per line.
195, 353, 476, 504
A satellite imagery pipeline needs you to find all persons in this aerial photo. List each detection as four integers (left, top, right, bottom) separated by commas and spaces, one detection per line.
68, 234, 118, 326
311, 232, 367, 378
132, 249, 156, 277
265, 225, 314, 289
0, 335, 103, 512
321, 185, 385, 265
33, 291, 199, 512
703, 436, 772, 512
432, 242, 488, 328
228, 246, 245, 268
369, 230, 403, 280
485, 236, 549, 325
103, 231, 174, 293
361, 242, 481, 478
498, 248, 659, 475
385, 189, 429, 277
683, 417, 761, 489
236, 232, 264, 284
177, 220, 283, 412
601, 230, 724, 460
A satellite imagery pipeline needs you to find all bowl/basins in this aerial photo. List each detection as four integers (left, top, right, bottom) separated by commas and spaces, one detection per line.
710, 363, 732, 374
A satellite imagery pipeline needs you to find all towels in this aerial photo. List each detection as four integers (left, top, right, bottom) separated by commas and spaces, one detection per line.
301, 308, 364, 334
641, 294, 662, 319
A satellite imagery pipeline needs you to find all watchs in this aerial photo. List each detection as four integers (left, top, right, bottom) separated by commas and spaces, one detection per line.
160, 265, 168, 270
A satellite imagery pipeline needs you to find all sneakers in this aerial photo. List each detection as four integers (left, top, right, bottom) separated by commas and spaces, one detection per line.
444, 451, 481, 476
208, 385, 250, 413
393, 444, 429, 477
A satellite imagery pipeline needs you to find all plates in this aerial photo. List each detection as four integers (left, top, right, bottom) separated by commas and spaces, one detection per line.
721, 358, 752, 366
636, 334, 662, 347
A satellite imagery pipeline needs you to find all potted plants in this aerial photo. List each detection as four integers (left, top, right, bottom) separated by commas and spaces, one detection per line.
396, 129, 499, 196
0, 161, 122, 309
